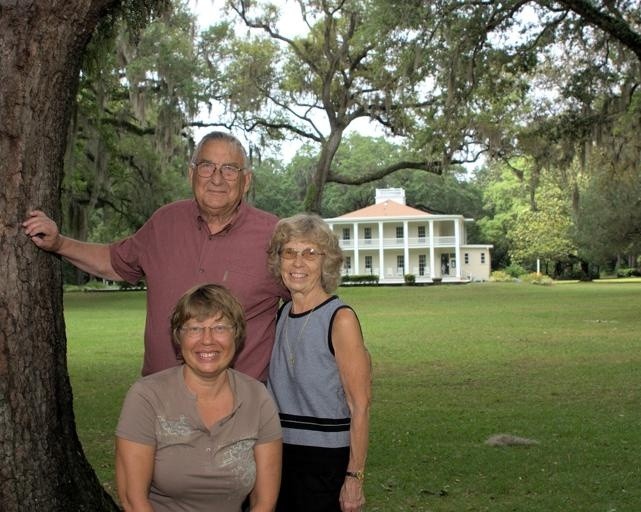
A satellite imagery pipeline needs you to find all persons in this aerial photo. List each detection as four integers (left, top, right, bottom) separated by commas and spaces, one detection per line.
114, 283, 284, 512
267, 212, 371, 512
23, 132, 292, 384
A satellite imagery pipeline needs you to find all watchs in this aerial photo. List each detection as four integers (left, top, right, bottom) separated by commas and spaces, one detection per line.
346, 471, 365, 478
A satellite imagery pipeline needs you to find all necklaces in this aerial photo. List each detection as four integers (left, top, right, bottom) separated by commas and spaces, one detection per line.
287, 296, 322, 364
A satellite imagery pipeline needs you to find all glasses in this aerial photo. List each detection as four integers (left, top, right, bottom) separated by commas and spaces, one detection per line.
196, 162, 244, 182
181, 321, 229, 338
279, 247, 325, 261
